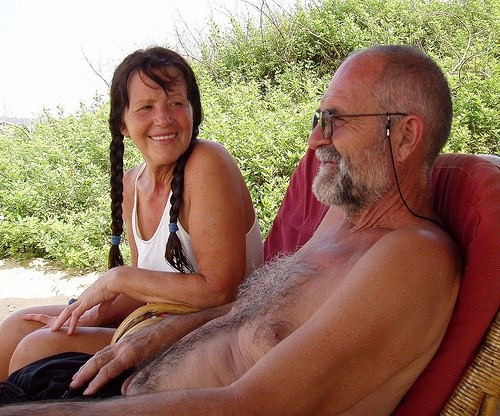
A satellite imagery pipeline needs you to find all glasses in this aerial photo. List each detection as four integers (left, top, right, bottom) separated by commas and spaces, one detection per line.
313, 111, 408, 140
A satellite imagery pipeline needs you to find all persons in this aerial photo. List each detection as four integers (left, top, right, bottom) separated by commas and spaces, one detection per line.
2, 44, 461, 414
0, 43, 266, 384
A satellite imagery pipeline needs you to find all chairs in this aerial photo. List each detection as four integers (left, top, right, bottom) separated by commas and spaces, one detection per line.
112, 147, 500, 416
